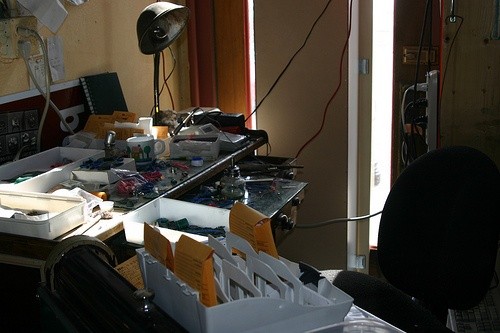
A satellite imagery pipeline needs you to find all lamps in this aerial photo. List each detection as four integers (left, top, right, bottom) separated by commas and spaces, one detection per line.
136, 2, 190, 125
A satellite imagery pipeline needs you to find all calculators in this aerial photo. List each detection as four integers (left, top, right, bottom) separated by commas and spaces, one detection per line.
199, 123, 250, 152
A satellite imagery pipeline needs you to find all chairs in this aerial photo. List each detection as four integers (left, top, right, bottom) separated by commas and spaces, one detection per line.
377, 146, 500, 333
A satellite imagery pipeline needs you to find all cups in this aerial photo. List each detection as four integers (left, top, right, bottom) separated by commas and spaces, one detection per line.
137, 117, 153, 135
99, 201, 114, 219
126, 136, 165, 165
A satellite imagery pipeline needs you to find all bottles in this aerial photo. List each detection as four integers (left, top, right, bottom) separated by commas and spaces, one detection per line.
131, 289, 166, 333
219, 166, 245, 202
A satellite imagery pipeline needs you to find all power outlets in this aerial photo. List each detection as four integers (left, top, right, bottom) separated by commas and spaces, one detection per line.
0, 16, 38, 59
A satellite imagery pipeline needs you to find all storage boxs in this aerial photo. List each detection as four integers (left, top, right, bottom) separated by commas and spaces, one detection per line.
0, 147, 105, 241
121, 195, 230, 245
169, 134, 220, 159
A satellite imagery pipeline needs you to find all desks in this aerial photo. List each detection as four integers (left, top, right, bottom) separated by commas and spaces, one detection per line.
0, 130, 307, 286
